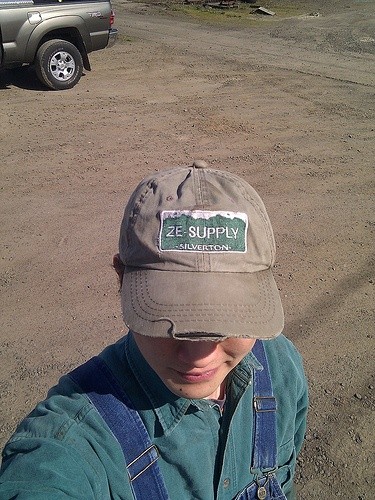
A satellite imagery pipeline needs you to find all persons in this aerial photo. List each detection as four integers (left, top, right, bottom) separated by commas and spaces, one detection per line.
0, 159, 308, 500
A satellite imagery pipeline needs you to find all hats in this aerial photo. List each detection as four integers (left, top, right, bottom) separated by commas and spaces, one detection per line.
117, 159, 284, 339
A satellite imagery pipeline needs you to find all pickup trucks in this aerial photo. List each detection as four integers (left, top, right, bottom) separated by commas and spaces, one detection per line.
0, 1, 118, 91
